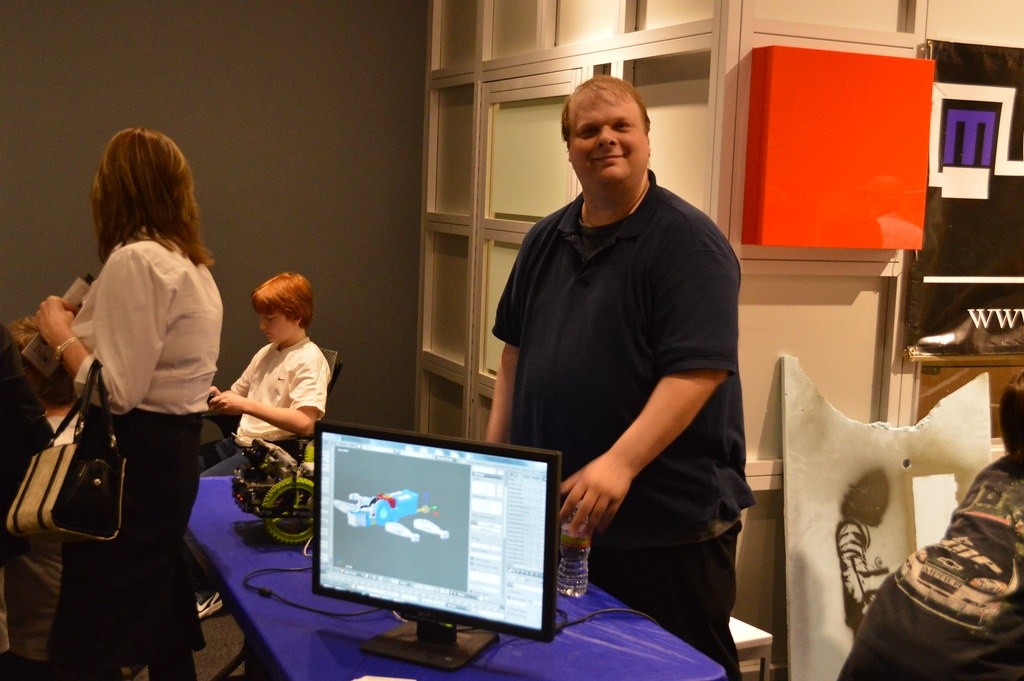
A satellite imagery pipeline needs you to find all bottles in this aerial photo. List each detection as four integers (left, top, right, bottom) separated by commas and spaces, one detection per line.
557, 500, 591, 597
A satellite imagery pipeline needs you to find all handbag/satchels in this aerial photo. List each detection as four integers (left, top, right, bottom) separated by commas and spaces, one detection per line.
7, 359, 127, 543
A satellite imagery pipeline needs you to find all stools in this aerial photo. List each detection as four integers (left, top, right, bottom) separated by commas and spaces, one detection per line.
728, 617, 773, 681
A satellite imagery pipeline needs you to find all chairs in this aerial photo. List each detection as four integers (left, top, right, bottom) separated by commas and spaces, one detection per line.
201, 345, 345, 464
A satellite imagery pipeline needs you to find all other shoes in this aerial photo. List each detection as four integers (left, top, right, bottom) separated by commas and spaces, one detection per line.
195, 588, 223, 619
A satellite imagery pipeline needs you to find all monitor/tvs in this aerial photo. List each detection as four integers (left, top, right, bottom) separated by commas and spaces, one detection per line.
311, 417, 562, 672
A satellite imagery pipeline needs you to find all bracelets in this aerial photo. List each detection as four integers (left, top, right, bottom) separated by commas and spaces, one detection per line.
54, 336, 80, 360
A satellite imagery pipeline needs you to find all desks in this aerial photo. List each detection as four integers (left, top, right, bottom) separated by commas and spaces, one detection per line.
187, 475, 727, 681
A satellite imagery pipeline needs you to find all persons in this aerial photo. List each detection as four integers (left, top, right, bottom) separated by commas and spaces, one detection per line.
179, 272, 333, 621
0, 128, 223, 681
836, 365, 1024, 681
484, 76, 755, 681
